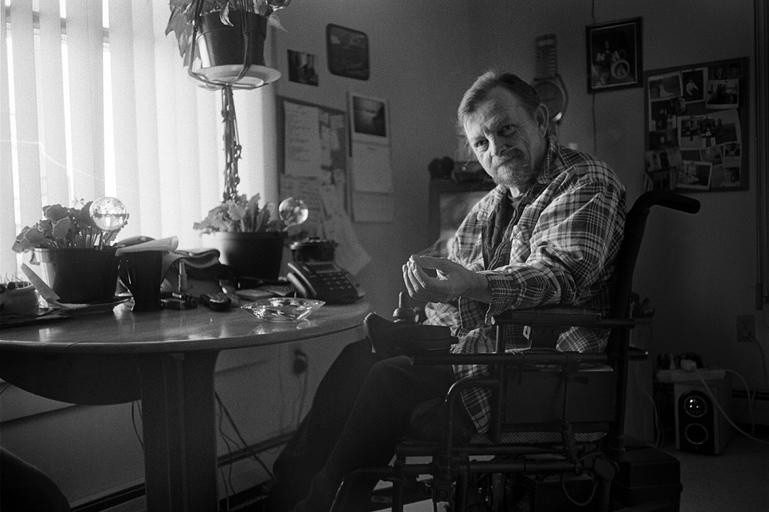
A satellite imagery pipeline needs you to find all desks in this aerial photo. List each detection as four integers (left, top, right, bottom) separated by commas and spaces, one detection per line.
0, 301, 369, 511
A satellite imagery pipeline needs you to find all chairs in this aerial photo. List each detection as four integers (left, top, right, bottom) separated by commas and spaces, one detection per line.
326, 188, 701, 511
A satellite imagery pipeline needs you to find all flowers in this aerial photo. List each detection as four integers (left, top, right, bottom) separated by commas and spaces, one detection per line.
9, 197, 134, 254
190, 194, 311, 237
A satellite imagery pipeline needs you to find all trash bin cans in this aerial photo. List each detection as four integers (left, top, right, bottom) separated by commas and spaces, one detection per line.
623, 347, 659, 450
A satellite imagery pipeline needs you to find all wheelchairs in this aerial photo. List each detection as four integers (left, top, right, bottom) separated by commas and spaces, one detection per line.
226, 183, 701, 512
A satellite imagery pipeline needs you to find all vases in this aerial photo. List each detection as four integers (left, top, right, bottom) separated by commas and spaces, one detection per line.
39, 244, 119, 301
219, 231, 285, 286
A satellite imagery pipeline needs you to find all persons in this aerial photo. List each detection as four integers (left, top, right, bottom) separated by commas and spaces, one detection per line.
648, 68, 743, 192
267, 68, 630, 510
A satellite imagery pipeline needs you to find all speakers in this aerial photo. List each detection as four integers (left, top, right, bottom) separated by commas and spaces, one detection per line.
674, 381, 734, 455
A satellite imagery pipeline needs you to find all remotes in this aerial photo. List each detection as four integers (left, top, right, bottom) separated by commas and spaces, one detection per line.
200, 290, 231, 311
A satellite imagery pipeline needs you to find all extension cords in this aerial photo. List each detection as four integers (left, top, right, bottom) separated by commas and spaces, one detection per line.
656, 367, 726, 382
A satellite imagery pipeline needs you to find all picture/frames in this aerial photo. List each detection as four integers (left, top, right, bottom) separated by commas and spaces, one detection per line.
641, 57, 751, 193
584, 15, 644, 94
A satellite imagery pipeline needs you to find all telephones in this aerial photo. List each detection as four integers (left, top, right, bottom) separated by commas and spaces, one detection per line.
286, 261, 367, 304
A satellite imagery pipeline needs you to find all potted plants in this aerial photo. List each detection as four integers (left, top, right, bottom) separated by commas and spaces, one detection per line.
162, 0, 289, 77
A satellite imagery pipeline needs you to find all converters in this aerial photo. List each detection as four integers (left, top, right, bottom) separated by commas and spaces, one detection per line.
681, 359, 697, 370
